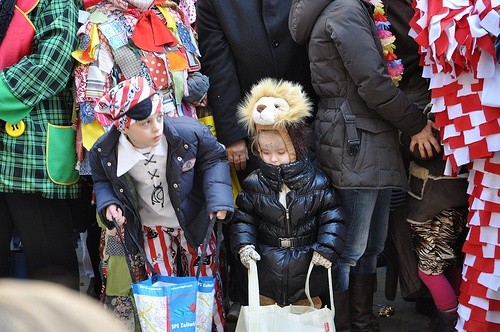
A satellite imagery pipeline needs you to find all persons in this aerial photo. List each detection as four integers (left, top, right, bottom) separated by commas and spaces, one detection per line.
196, 0, 320, 187
289, 0, 469, 332
70, 0, 210, 332
231, 78, 347, 310
89, 79, 234, 332
0, 0, 82, 290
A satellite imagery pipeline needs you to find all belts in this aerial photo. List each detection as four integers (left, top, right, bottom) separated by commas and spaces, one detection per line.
265, 233, 314, 249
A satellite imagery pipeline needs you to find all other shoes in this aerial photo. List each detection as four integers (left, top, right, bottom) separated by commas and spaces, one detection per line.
421, 312, 458, 332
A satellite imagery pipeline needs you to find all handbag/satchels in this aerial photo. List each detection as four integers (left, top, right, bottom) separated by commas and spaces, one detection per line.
236, 256, 339, 332
109, 211, 220, 332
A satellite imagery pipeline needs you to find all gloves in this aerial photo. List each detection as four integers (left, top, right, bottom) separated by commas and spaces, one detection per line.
238, 244, 261, 268
312, 251, 333, 269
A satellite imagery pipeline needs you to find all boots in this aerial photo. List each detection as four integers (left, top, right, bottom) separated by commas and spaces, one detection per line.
350, 271, 382, 332
335, 301, 350, 332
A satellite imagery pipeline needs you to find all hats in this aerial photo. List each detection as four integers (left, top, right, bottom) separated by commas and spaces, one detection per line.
95, 77, 163, 128
